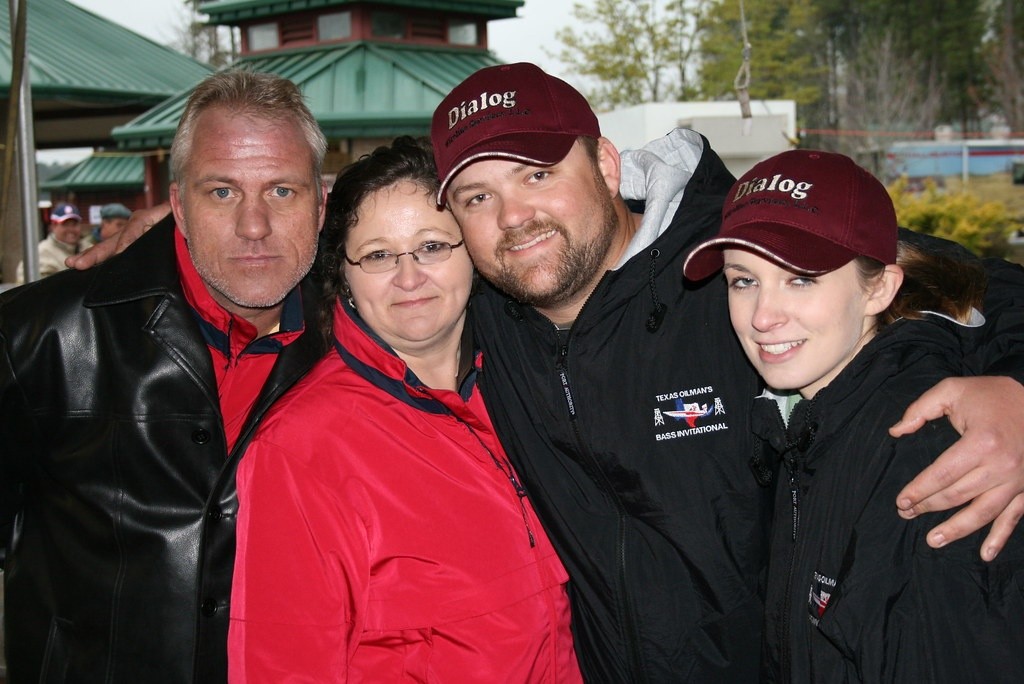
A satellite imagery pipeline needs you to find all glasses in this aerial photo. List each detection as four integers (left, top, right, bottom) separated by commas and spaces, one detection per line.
344, 239, 465, 274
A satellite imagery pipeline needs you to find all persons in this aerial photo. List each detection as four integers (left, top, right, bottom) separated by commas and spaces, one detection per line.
683, 148, 1023, 684
63, 63, 1022, 684
1, 69, 331, 684
14, 201, 130, 283
226, 134, 583, 684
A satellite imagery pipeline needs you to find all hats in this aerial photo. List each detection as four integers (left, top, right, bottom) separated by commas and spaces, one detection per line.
683, 150, 899, 280
431, 62, 601, 212
50, 203, 82, 223
100, 203, 131, 219
